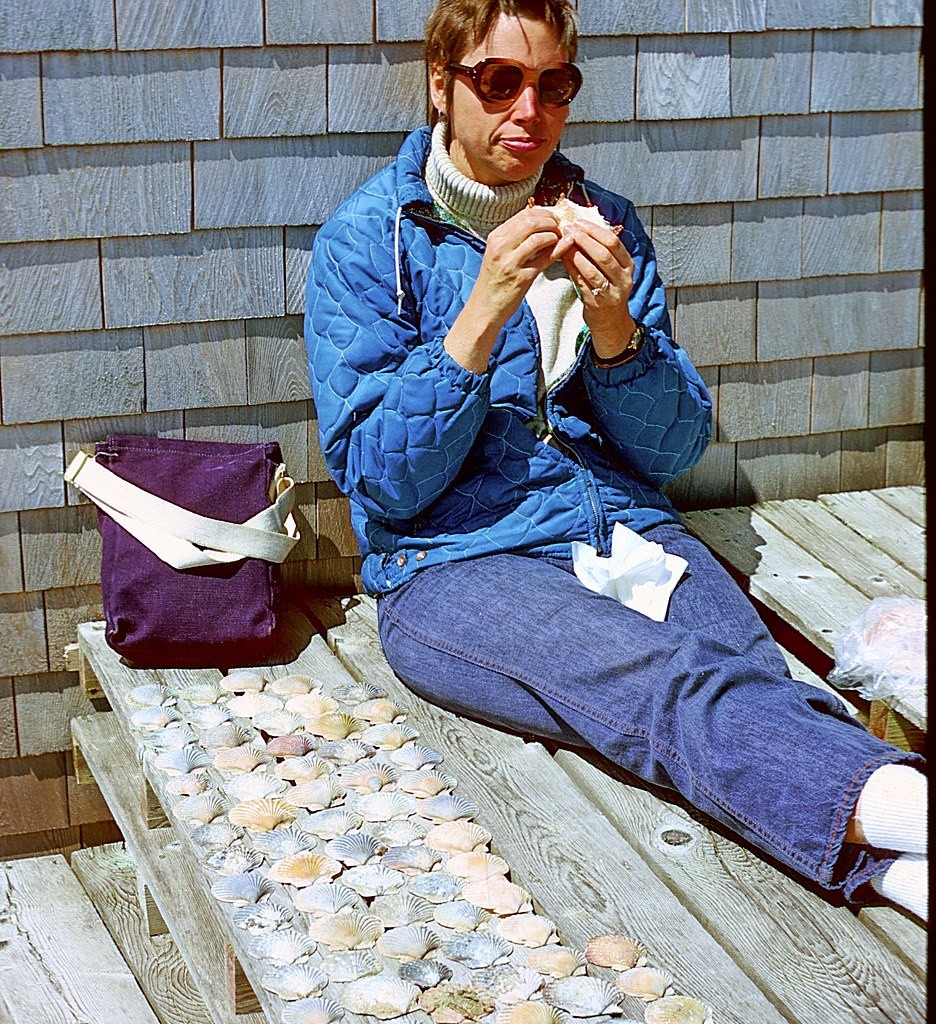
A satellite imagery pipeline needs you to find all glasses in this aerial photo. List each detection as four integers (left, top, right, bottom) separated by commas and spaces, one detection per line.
445, 58, 582, 109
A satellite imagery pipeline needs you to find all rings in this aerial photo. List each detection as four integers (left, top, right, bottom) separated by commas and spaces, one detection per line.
591, 281, 608, 295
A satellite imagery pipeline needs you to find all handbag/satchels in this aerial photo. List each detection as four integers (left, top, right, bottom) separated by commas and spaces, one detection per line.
63, 433, 301, 660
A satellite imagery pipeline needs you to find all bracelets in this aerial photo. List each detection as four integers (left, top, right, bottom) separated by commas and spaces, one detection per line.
591, 318, 642, 364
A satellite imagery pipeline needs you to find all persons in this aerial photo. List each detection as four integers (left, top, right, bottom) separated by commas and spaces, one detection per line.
302, 1, 929, 928
